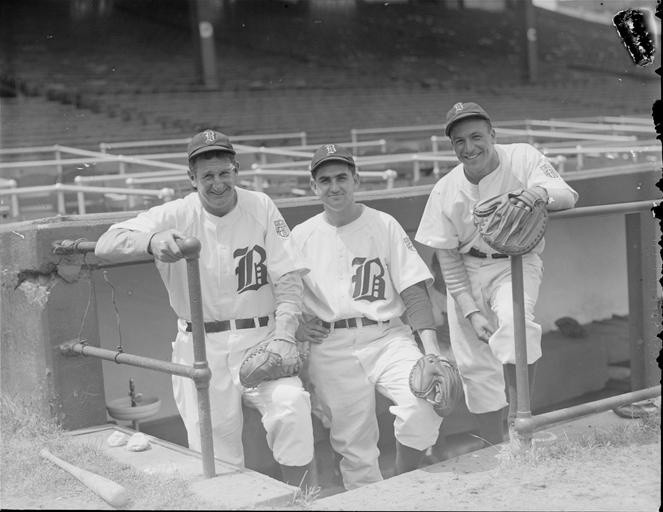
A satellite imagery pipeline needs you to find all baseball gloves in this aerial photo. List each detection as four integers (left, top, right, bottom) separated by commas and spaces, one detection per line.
409, 354, 462, 416
473, 189, 548, 254
240, 340, 303, 388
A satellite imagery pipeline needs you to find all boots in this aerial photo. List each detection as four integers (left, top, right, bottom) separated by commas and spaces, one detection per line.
393, 440, 428, 475
472, 363, 535, 455
278, 464, 311, 493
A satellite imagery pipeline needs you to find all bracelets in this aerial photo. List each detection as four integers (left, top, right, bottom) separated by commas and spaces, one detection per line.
535, 183, 552, 208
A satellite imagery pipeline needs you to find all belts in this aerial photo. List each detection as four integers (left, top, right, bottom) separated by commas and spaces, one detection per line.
186, 316, 269, 333
468, 247, 508, 262
323, 317, 390, 328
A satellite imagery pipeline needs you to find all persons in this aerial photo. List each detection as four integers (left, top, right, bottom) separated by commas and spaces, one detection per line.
414, 102, 579, 445
94, 130, 317, 490
291, 146, 463, 490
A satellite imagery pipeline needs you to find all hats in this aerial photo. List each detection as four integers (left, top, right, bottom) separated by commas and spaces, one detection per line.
187, 129, 236, 160
311, 144, 354, 173
446, 101, 489, 136
